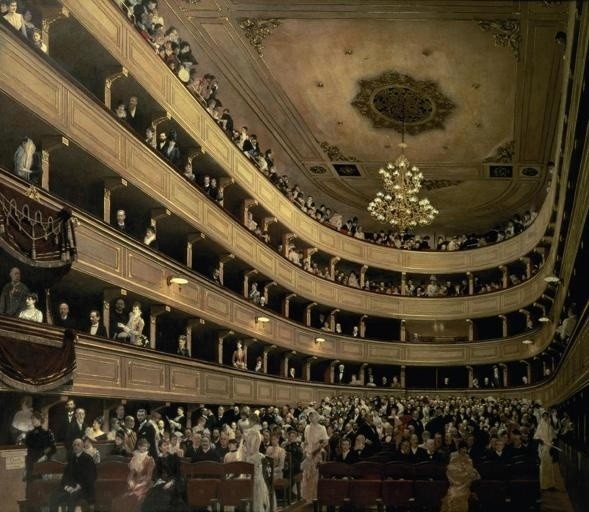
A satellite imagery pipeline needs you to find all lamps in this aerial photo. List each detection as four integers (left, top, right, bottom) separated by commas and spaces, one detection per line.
367, 100, 439, 244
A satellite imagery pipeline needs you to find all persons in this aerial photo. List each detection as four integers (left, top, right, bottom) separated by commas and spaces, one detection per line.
1, 2, 588, 386
10, 394, 589, 511
